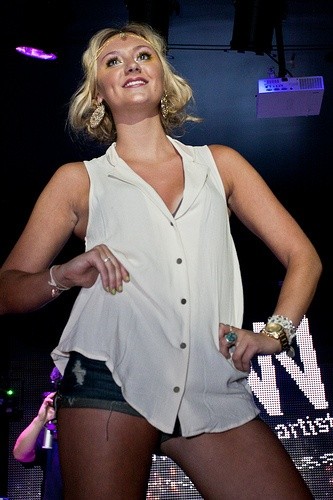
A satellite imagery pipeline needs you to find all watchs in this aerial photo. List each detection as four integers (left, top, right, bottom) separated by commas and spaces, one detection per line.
260, 323, 289, 355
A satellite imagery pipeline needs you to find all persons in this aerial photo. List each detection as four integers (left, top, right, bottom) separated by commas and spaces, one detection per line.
0, 24, 322, 500
13, 392, 63, 500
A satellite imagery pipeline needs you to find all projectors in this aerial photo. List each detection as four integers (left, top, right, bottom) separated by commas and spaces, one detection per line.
255, 76, 324, 119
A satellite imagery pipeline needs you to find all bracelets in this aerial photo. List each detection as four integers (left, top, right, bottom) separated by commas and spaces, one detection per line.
267, 314, 296, 345
48, 265, 71, 298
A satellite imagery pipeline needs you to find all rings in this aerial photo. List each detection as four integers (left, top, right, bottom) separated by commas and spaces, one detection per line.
227, 324, 232, 332
103, 257, 110, 262
225, 332, 238, 345
